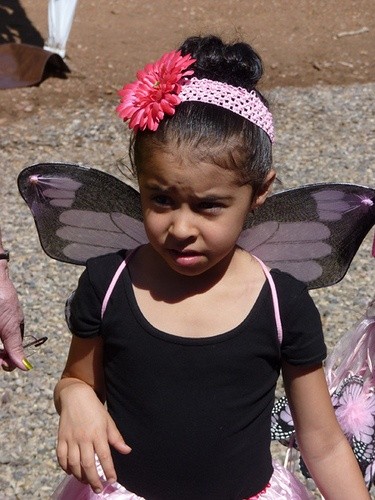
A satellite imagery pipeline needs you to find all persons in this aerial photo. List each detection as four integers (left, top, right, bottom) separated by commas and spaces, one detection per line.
0, 228, 32, 372
53, 35, 375, 500
272, 234, 375, 500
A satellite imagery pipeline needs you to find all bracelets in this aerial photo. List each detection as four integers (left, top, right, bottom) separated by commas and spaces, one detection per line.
0, 249, 10, 262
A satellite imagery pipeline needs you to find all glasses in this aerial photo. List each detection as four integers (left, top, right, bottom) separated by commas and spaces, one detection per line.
0, 335, 48, 359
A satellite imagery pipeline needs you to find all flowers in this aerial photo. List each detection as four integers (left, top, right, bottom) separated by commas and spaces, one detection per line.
114, 50, 197, 132
